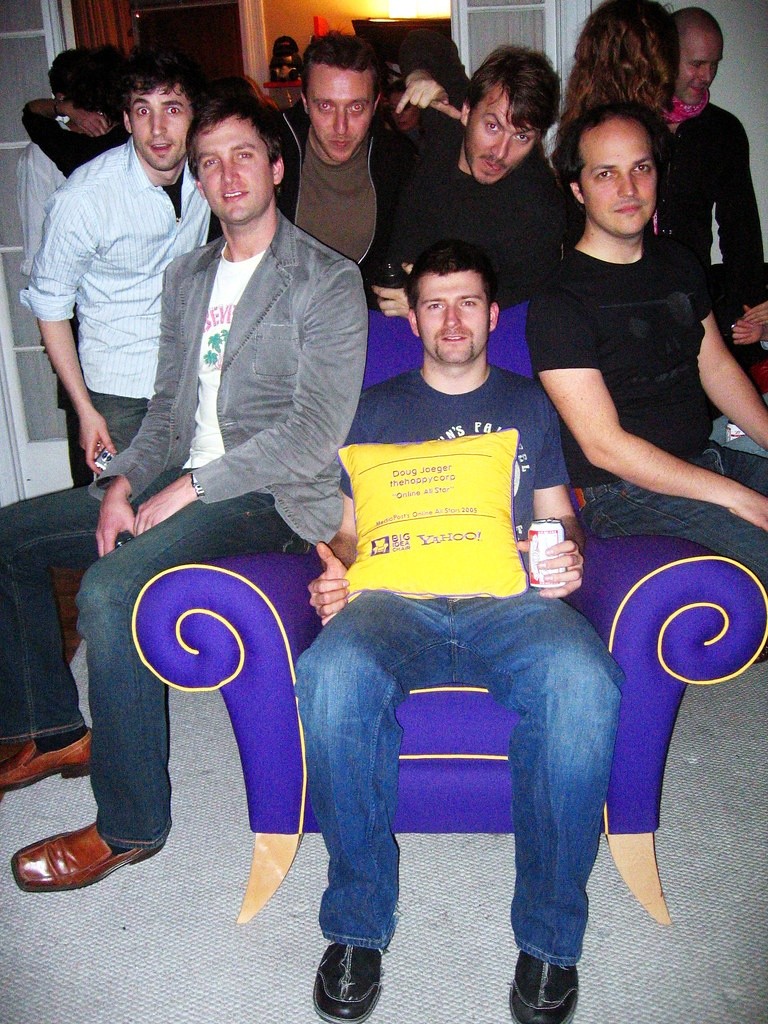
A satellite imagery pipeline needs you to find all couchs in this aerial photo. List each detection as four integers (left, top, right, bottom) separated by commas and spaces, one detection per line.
133, 307, 767, 927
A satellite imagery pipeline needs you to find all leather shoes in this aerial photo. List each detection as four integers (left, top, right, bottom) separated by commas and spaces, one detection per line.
0, 727, 91, 792
510, 949, 578, 1024
11, 823, 165, 893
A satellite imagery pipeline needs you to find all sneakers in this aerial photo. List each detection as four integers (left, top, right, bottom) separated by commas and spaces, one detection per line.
313, 942, 381, 1024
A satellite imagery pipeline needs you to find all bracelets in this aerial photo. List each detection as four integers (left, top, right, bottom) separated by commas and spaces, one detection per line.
53, 100, 66, 117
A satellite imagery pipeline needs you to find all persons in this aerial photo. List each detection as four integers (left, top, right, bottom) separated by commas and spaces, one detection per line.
16, 0, 768, 346
0, 94, 369, 893
527, 102, 768, 589
295, 233, 627, 1024
18, 49, 212, 476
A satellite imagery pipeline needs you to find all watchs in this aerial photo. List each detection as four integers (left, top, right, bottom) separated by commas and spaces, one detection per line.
191, 474, 206, 496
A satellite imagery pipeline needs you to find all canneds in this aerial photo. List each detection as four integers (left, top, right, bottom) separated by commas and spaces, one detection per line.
527, 517, 568, 588
